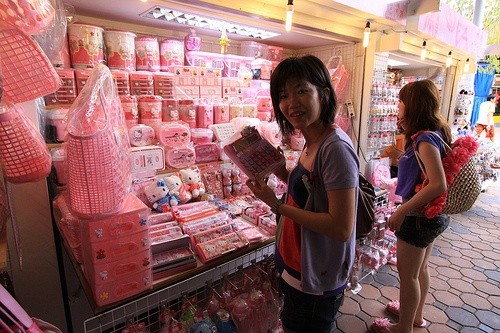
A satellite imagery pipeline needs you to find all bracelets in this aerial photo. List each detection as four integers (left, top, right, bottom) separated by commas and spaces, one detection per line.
270, 200, 285, 215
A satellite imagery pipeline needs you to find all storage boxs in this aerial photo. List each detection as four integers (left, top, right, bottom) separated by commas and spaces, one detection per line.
240, 41, 269, 59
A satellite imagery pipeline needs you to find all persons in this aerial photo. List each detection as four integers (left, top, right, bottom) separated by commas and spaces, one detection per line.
380, 131, 406, 179
239, 54, 362, 333
476, 93, 496, 142
370, 79, 451, 333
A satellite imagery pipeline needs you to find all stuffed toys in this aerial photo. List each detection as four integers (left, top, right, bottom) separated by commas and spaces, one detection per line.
143, 164, 206, 212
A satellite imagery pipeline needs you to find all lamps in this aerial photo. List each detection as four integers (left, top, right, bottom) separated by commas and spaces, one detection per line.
421, 41, 427, 60
363, 21, 370, 47
464, 58, 470, 72
445, 51, 452, 67
286, 0, 294, 31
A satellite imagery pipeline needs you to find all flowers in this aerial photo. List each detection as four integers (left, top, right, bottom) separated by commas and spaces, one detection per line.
415, 134, 480, 218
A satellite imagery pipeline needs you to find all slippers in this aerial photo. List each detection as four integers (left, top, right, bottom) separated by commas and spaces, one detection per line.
373, 317, 415, 333
387, 300, 426, 327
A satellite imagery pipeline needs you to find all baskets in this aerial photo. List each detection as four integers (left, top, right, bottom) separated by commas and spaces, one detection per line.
0, 100, 52, 184
67, 64, 132, 218
0, 19, 63, 103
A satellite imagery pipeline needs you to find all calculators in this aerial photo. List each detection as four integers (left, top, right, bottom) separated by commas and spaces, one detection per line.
223, 130, 286, 183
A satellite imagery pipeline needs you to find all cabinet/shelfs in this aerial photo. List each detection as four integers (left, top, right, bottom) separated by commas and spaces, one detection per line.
80, 191, 152, 308
46, 69, 243, 103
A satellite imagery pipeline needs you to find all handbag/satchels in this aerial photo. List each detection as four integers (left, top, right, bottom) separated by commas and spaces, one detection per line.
411, 130, 481, 219
356, 172, 376, 234
